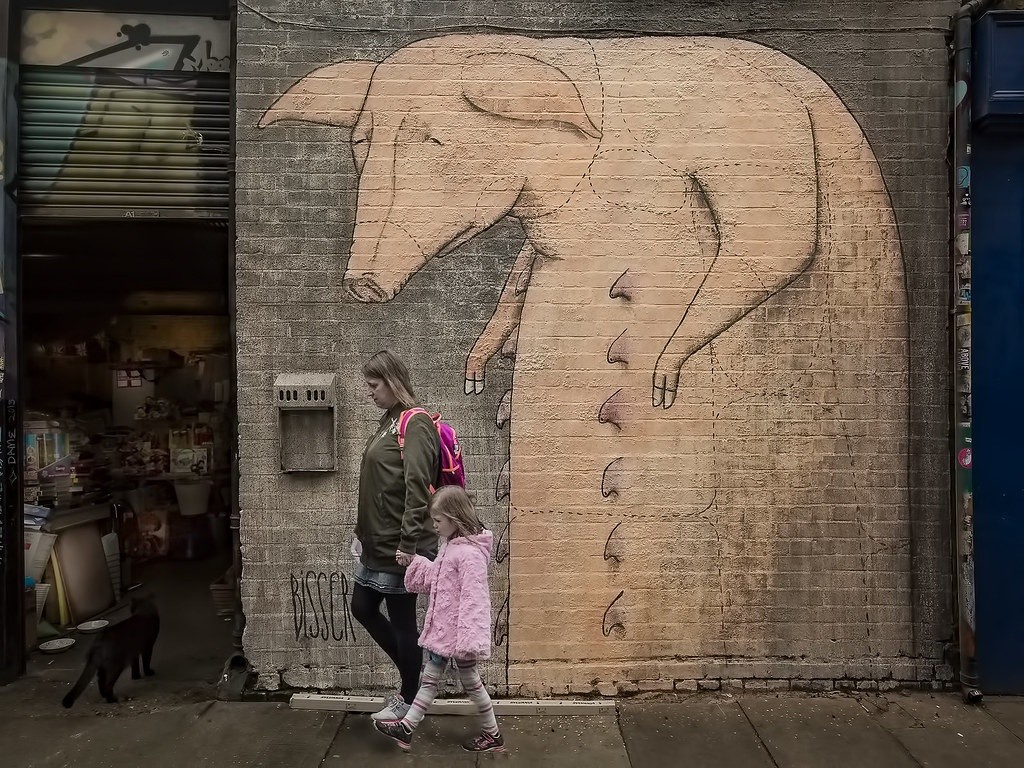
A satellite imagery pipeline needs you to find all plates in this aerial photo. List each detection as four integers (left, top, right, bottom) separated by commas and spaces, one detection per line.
77, 620, 109, 633
39, 638, 75, 652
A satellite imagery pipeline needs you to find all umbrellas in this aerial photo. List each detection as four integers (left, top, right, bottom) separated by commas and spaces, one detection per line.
101, 504, 121, 603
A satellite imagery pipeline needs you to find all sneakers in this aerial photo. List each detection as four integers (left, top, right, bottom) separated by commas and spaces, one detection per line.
373, 720, 413, 749
460, 731, 506, 752
370, 694, 425, 723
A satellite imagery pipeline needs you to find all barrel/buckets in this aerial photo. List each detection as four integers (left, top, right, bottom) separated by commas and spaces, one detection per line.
211, 585, 235, 609
175, 484, 211, 515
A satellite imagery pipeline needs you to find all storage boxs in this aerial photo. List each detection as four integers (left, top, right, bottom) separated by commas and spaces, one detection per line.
23, 458, 106, 656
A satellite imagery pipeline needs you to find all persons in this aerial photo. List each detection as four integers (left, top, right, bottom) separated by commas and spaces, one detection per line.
373, 485, 506, 751
352, 349, 442, 724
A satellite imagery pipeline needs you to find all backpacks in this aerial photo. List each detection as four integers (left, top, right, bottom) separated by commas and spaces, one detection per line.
397, 407, 466, 495
